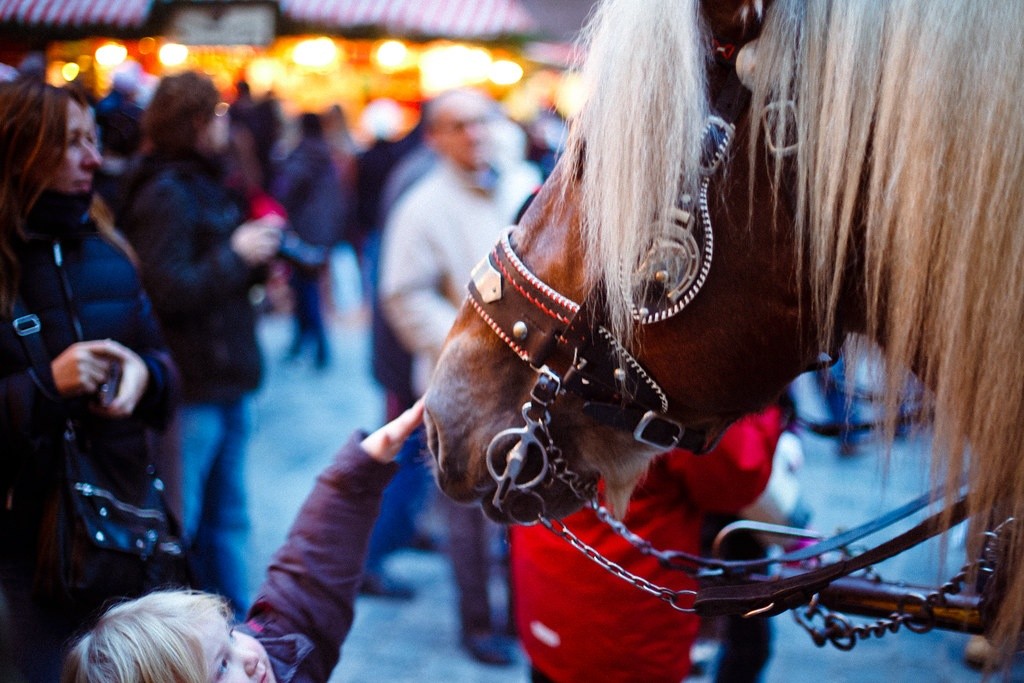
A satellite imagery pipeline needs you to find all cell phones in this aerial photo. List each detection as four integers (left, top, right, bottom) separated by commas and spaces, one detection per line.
99, 361, 123, 403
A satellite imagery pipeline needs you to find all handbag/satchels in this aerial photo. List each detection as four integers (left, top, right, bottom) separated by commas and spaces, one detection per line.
31, 435, 192, 632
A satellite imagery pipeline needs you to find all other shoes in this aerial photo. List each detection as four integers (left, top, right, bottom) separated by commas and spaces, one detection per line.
405, 530, 449, 550
466, 628, 517, 671
354, 572, 415, 602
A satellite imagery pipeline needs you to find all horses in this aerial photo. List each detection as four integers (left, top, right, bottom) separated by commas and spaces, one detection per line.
421, 1, 1023, 683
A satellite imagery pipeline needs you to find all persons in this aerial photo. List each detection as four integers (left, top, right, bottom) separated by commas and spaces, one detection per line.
352, 251, 442, 600
120, 63, 277, 625
507, 347, 864, 683
65, 382, 438, 682
375, 87, 529, 664
1, 71, 187, 682
225, 79, 586, 365
